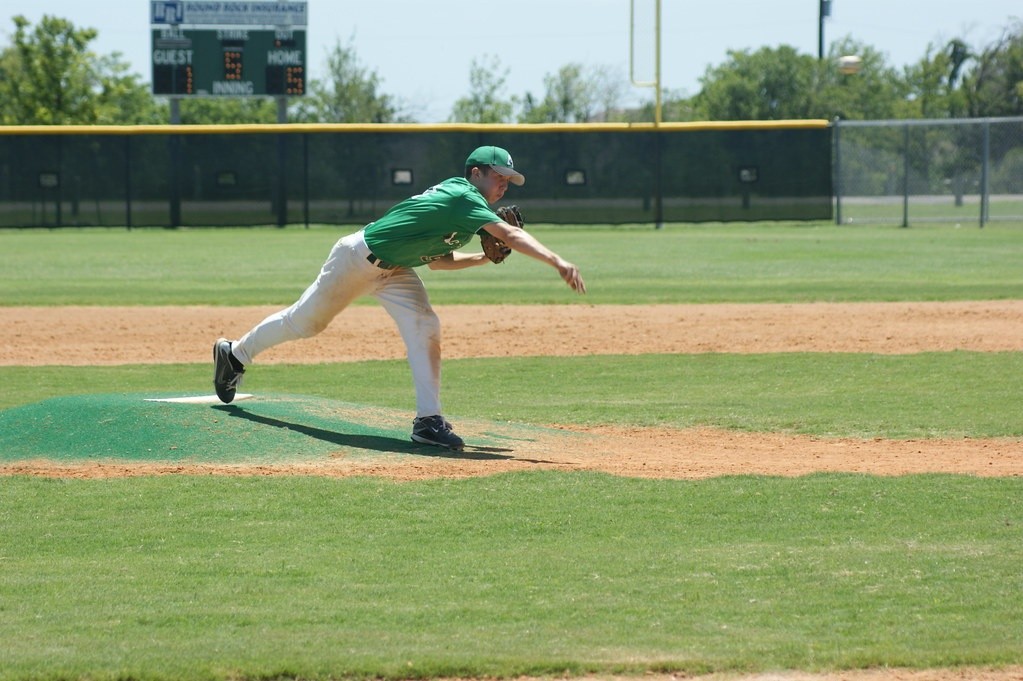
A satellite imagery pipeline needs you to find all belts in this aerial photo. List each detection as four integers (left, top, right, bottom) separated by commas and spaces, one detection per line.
366, 253, 397, 270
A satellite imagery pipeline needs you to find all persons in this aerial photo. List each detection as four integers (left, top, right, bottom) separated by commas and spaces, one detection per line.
213, 145, 587, 448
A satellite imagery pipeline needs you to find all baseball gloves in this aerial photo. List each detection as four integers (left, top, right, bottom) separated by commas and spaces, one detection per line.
480, 205, 525, 263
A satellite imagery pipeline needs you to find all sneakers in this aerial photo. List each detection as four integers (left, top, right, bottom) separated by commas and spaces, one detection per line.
213, 338, 246, 404
411, 415, 465, 449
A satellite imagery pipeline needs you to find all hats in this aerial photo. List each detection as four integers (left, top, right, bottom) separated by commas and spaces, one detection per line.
466, 146, 525, 186
